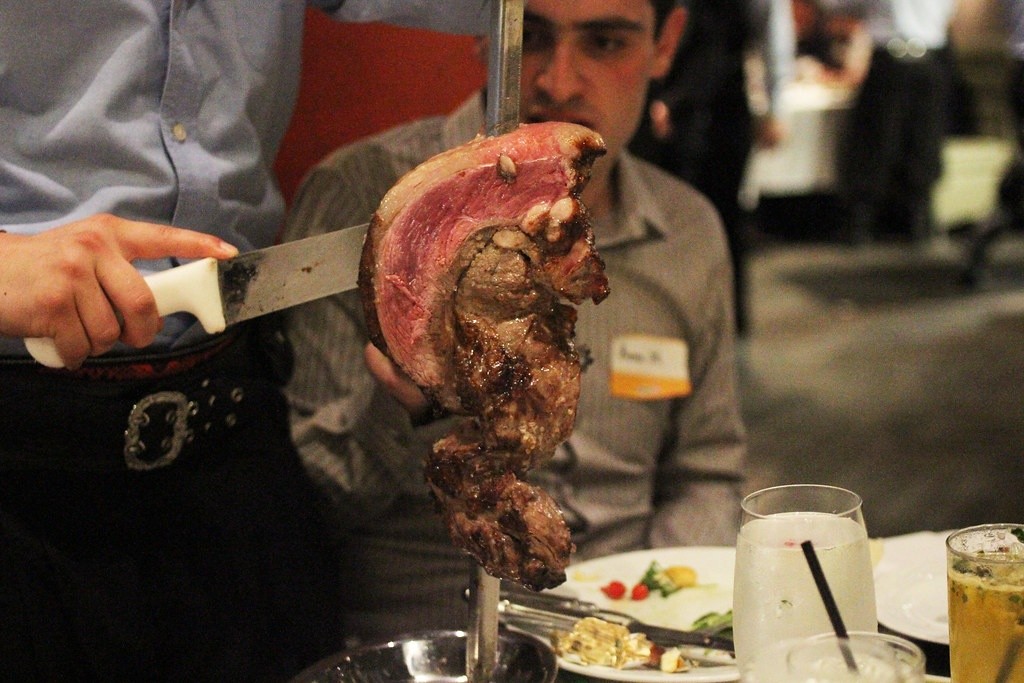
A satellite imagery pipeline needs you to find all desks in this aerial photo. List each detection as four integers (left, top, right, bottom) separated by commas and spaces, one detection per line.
739, 81, 852, 209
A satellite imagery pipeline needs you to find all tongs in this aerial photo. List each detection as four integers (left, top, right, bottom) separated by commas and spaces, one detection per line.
465, 578, 740, 667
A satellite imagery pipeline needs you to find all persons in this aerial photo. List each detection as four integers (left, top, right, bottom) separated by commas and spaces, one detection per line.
0, 0, 493, 683
281, 0, 746, 649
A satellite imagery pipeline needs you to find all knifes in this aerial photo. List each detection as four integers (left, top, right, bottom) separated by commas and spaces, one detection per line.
24, 223, 374, 369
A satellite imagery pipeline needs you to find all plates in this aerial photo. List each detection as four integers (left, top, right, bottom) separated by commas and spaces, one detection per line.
497, 546, 746, 683
874, 532, 953, 647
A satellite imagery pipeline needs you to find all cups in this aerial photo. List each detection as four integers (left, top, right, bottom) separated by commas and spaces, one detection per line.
731, 483, 876, 683
787, 632, 924, 683
945, 523, 1024, 683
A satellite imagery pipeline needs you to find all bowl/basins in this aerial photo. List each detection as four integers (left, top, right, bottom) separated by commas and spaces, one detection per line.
289, 629, 558, 683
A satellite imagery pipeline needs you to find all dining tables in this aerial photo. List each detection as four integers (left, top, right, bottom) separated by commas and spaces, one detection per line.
283, 531, 1024, 683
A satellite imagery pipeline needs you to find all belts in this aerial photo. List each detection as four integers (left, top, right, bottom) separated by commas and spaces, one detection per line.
0, 347, 280, 474
